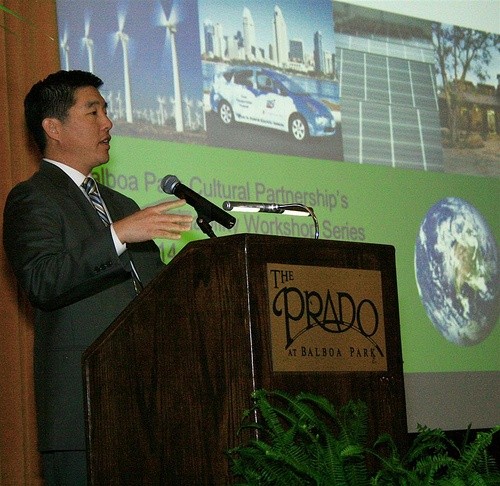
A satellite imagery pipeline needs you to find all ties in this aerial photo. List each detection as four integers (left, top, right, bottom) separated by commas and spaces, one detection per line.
80, 178, 111, 233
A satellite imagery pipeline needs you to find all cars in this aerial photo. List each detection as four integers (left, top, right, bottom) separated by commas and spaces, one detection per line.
210, 66, 337, 141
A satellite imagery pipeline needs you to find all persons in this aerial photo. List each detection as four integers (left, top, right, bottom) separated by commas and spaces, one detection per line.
6, 68, 195, 486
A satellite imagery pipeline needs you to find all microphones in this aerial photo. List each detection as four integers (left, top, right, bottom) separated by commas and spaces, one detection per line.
160, 174, 236, 232
221, 198, 313, 218
